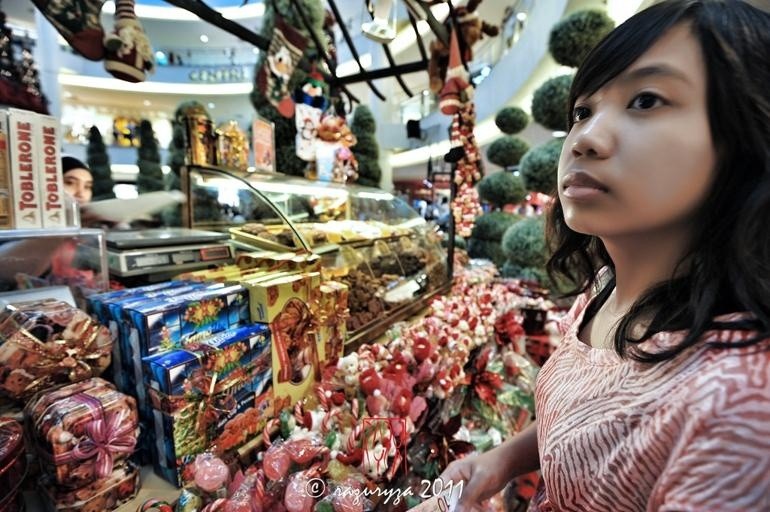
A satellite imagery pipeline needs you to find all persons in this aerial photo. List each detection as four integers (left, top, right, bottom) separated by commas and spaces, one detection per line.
431, 0, 769, 512
1, 157, 132, 286
